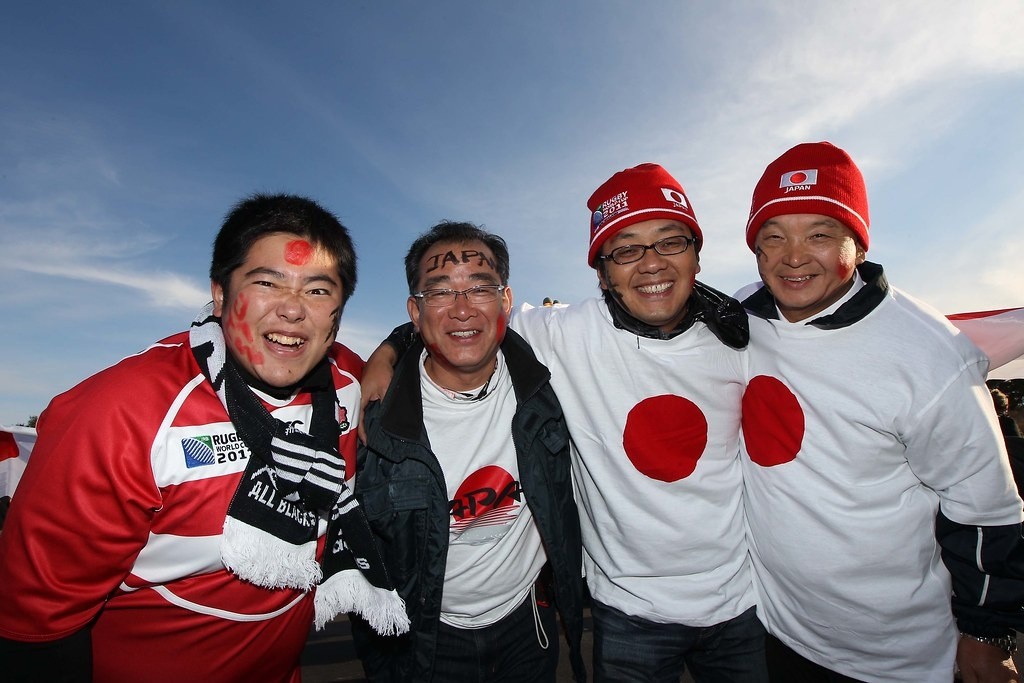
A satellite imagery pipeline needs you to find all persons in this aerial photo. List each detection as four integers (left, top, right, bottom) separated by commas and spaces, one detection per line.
726, 139, 1024, 682
342, 219, 589, 683
0, 192, 365, 683
356, 162, 769, 683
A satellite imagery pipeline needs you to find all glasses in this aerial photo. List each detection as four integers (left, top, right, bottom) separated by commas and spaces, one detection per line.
414, 284, 506, 307
599, 235, 696, 265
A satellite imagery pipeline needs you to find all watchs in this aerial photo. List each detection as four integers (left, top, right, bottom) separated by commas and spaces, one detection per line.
961, 631, 1019, 656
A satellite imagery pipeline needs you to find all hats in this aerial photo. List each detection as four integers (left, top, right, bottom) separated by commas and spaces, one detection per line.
745, 141, 869, 254
587, 162, 703, 270
